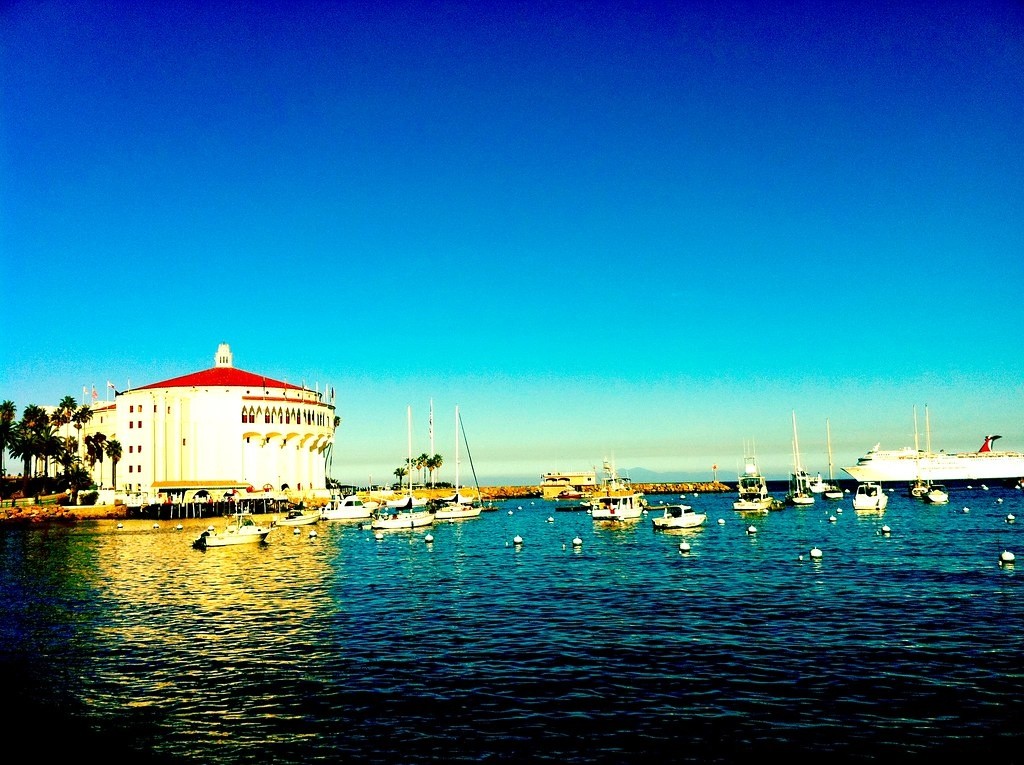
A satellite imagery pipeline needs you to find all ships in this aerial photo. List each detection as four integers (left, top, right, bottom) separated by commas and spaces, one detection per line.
842, 435, 1024, 484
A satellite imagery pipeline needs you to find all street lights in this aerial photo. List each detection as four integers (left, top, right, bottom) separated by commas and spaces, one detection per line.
68, 481, 73, 504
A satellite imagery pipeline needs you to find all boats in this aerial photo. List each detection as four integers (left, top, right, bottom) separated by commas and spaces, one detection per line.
204, 512, 278, 547
553, 489, 593, 498
479, 505, 498, 511
852, 481, 888, 510
651, 505, 707, 530
580, 452, 651, 507
810, 472, 837, 494
277, 509, 320, 527
590, 495, 643, 518
536, 472, 596, 499
322, 497, 376, 519
732, 435, 773, 511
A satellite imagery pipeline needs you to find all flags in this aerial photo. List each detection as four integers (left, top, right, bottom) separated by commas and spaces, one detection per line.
84, 386, 89, 395
108, 380, 115, 389
92, 385, 98, 399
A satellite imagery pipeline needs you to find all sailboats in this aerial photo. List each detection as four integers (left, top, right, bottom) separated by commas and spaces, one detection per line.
786, 408, 815, 504
434, 405, 482, 522
823, 416, 847, 498
923, 402, 949, 504
909, 404, 930, 497
372, 407, 434, 531
